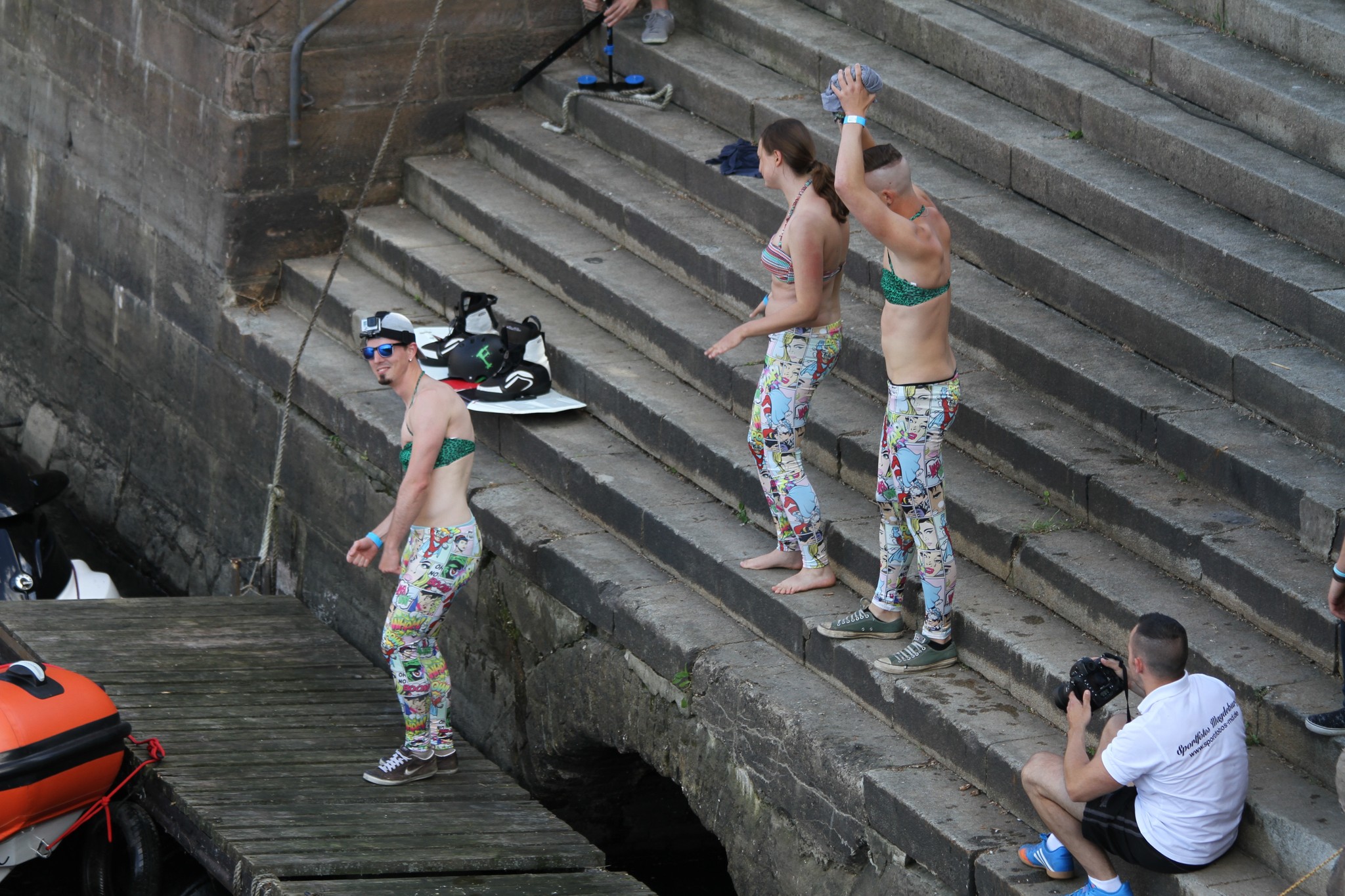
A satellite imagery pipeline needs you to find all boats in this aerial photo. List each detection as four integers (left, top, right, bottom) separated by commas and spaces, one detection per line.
0, 660, 131, 884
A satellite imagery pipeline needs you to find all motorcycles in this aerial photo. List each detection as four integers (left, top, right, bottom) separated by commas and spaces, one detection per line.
0, 468, 125, 600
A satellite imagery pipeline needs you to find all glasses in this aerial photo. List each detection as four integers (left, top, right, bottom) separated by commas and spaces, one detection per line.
359, 342, 408, 360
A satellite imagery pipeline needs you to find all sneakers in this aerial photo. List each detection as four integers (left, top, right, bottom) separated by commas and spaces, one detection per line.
1018, 833, 1074, 880
420, 291, 499, 366
1065, 878, 1133, 896
362, 744, 458, 786
474, 315, 551, 402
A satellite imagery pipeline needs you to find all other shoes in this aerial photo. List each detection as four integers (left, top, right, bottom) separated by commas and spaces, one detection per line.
816, 598, 906, 640
875, 631, 958, 673
1305, 707, 1345, 736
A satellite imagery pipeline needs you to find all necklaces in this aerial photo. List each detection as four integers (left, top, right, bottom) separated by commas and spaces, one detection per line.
920, 208, 928, 217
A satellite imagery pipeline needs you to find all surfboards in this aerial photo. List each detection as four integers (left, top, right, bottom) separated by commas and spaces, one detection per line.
414, 327, 588, 415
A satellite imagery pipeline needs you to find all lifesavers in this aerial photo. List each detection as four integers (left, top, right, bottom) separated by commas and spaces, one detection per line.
85, 798, 161, 895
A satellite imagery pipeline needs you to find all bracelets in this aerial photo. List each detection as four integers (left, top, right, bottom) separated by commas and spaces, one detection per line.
1333, 563, 1345, 583
366, 531, 384, 549
843, 115, 865, 127
763, 294, 769, 306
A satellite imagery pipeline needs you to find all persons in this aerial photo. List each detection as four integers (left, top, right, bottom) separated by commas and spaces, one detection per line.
816, 63, 963, 674
347, 310, 483, 786
703, 118, 852, 595
1018, 609, 1249, 896
583, 0, 675, 45
1305, 537, 1345, 736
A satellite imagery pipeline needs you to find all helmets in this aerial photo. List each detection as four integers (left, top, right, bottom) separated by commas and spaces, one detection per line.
447, 334, 504, 384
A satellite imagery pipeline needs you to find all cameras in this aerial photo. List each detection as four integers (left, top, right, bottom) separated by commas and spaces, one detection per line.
1053, 657, 1125, 714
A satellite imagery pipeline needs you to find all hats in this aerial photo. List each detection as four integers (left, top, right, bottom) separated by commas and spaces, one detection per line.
360, 311, 428, 361
